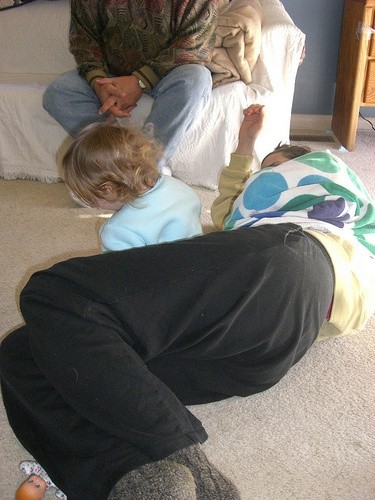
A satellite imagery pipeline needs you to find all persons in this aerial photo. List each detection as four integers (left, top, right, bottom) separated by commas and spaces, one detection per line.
61, 122, 202, 253
15, 458, 67, 500
41, 0, 219, 172
1, 104, 375, 500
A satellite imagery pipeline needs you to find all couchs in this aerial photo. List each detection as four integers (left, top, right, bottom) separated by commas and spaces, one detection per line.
0, 0, 311, 192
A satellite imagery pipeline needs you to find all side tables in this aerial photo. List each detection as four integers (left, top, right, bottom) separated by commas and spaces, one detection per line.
328, 0, 375, 153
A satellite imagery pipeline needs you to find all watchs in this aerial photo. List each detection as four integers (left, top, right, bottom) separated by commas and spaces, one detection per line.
138, 80, 146, 89
